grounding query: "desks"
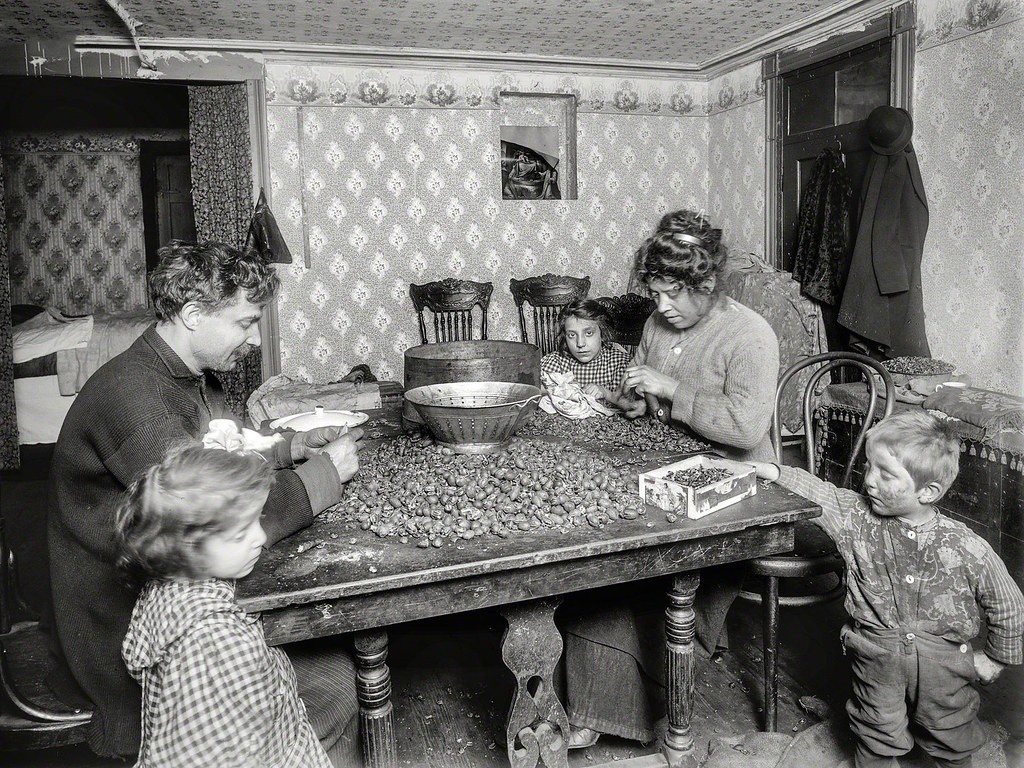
[235,402,822,768]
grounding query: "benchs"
[719,249,828,441]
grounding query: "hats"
[866,105,913,156]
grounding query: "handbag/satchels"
[242,187,292,266]
[501,140,562,200]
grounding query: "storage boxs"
[637,453,756,520]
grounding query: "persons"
[46,239,365,768]
[539,210,779,750]
[113,442,332,768]
[742,408,1024,768]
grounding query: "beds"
[10,305,160,445]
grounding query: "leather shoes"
[555,724,601,749]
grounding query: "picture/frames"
[499,91,578,200]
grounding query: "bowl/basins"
[402,381,541,455]
[881,358,956,396]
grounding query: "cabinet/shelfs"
[822,382,1024,598]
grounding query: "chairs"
[509,273,591,357]
[409,278,493,344]
[737,351,895,731]
[0,515,94,752]
[594,293,657,346]
